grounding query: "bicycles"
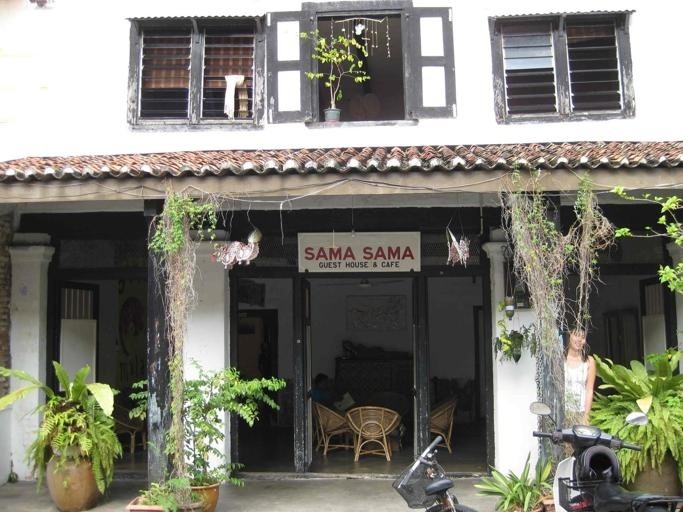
[391,434,479,512]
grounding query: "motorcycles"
[530,401,682,512]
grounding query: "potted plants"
[300,28,372,121]
[472,457,553,512]
[589,348,683,496]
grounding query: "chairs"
[312,396,458,461]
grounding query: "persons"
[308,373,353,440]
[561,322,598,459]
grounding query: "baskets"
[557,477,607,512]
[392,458,447,509]
